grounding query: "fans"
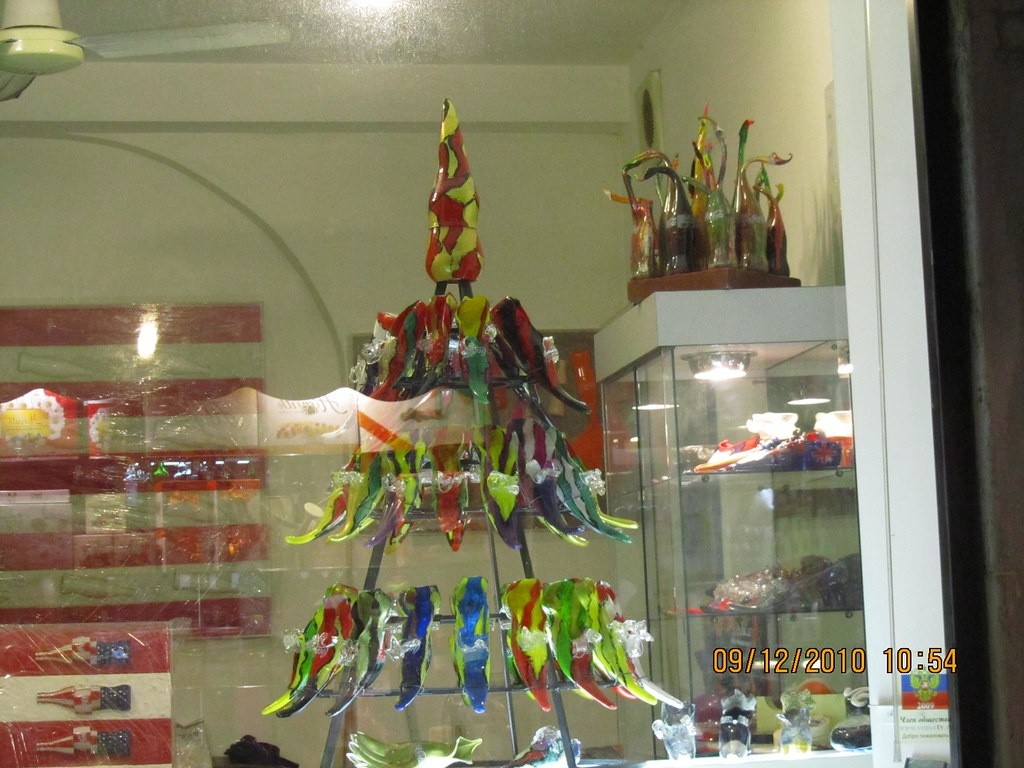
[0,0,289,105]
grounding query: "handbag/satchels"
[224,734,299,768]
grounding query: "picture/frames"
[347,328,607,532]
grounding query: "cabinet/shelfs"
[589,283,875,768]
